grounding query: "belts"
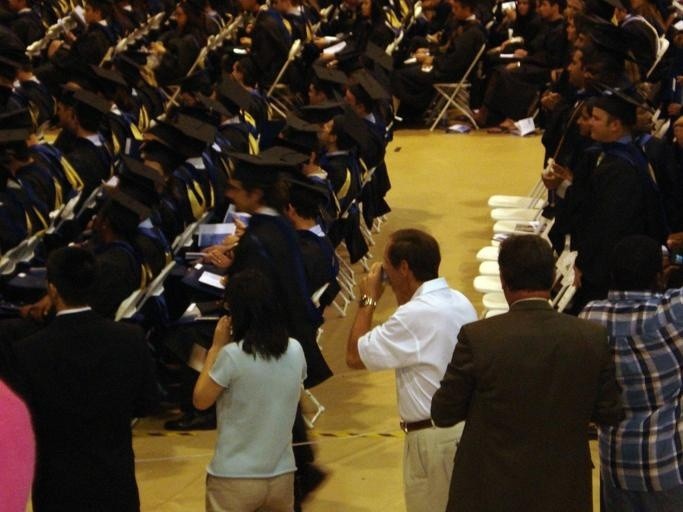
[400,414,434,433]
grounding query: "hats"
[573,0,655,126]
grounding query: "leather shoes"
[164,411,217,431]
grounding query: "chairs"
[473,176,578,320]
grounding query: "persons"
[575,236,683,511]
[390,0,528,133]
[0,2,394,512]
[530,1,682,434]
[342,228,480,512]
[429,234,624,511]
